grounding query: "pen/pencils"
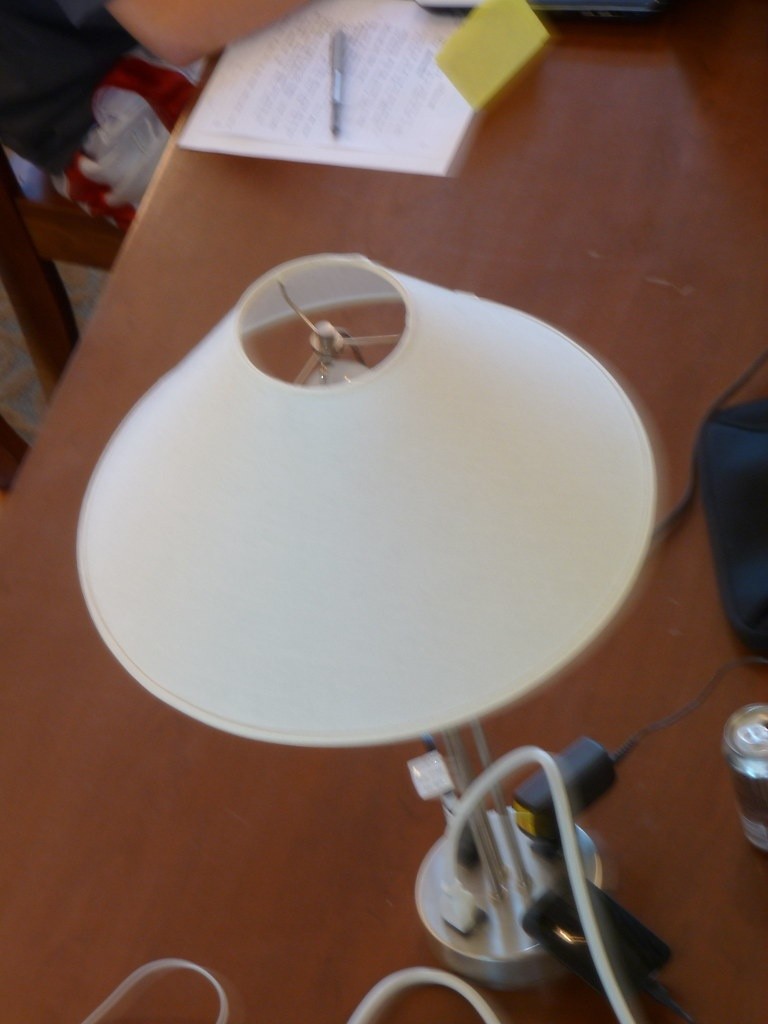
[329,30,345,139]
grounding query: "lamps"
[73,248,662,990]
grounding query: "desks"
[0,0,768,1024]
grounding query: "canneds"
[722,703,768,853]
